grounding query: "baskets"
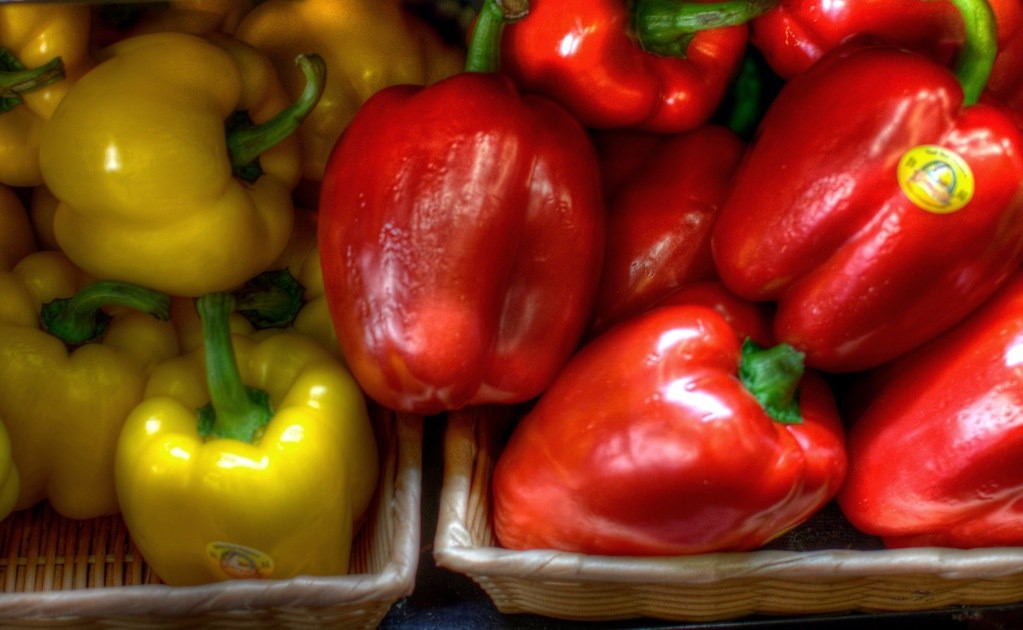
[0,401,422,630]
[432,401,1023,618]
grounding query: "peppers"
[0,0,1023,588]
